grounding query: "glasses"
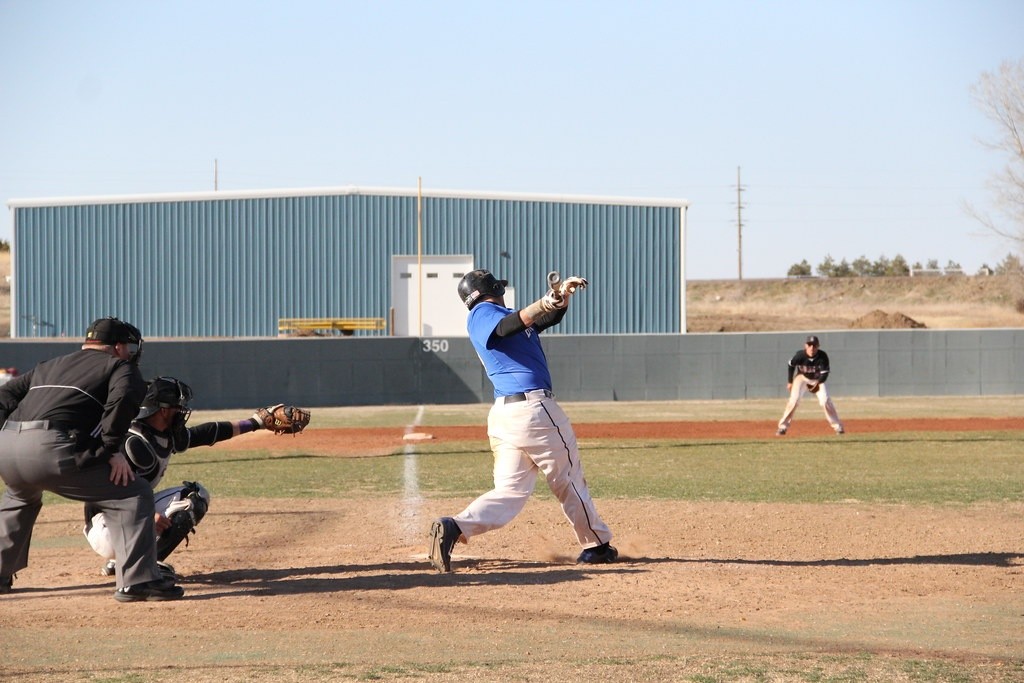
[807,342,819,348]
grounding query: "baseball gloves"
[255,402,312,435]
[806,383,819,393]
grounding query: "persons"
[1,317,183,603]
[83,376,311,577]
[776,336,844,435]
[430,270,619,571]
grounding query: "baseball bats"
[547,271,561,299]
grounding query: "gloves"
[542,289,563,316]
[557,274,588,299]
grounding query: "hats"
[86,318,143,345]
[134,375,190,419]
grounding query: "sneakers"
[0,576,14,595]
[153,561,175,578]
[103,559,116,576]
[429,517,460,573]
[114,580,186,603]
[577,541,619,567]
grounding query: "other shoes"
[835,428,845,436]
[776,428,785,436]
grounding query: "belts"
[500,390,552,404]
[3,420,70,437]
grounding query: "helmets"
[805,336,820,344]
[456,269,509,310]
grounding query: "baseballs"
[715,295,723,301]
